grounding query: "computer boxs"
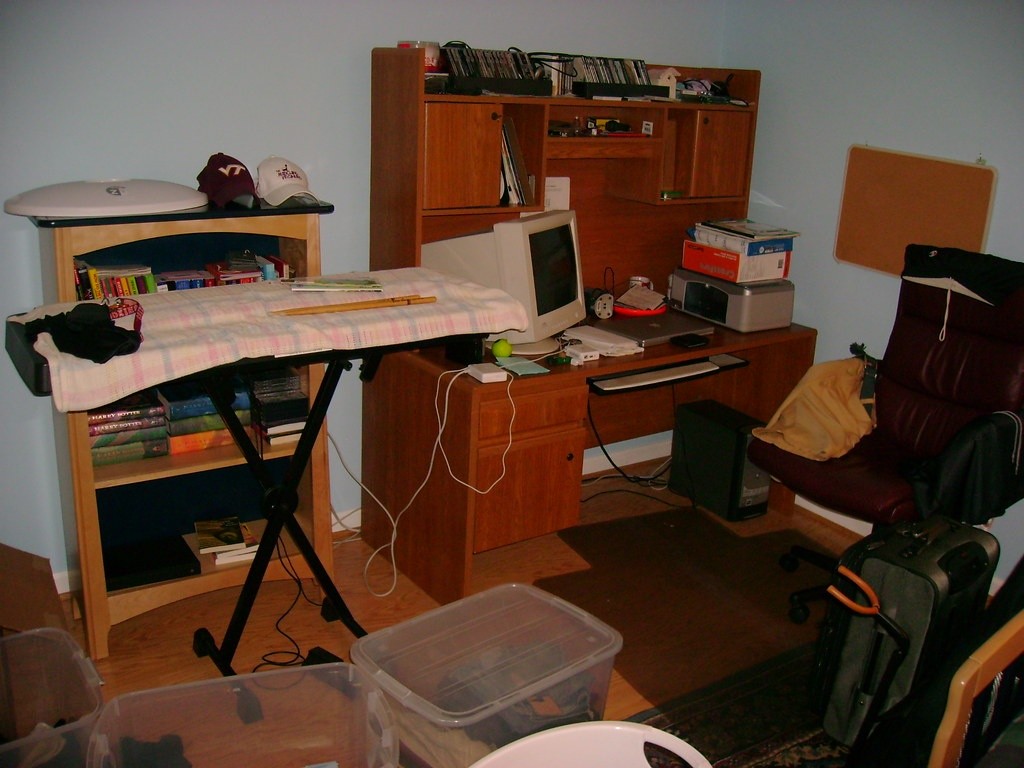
[668,398,773,523]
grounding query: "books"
[194,519,259,566]
[88,393,168,467]
[424,73,449,76]
[682,217,801,283]
[74,254,296,301]
[291,277,383,292]
[499,115,535,207]
[592,96,622,101]
[440,47,536,79]
[570,55,651,86]
[155,361,310,456]
[624,94,673,102]
[550,54,572,97]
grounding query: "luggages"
[824,516,1000,756]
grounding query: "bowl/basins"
[397,40,442,72]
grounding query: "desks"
[6,267,527,722]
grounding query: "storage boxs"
[0,626,106,768]
[84,660,400,768]
[349,584,625,767]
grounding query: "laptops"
[593,310,715,347]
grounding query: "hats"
[256,155,320,206]
[197,152,262,205]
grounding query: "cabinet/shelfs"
[22,197,334,667]
[358,44,821,604]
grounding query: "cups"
[629,276,653,291]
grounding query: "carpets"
[537,504,833,713]
[613,639,929,768]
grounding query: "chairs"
[753,243,1024,542]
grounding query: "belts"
[859,351,877,417]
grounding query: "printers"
[669,265,795,333]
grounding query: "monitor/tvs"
[420,210,586,353]
